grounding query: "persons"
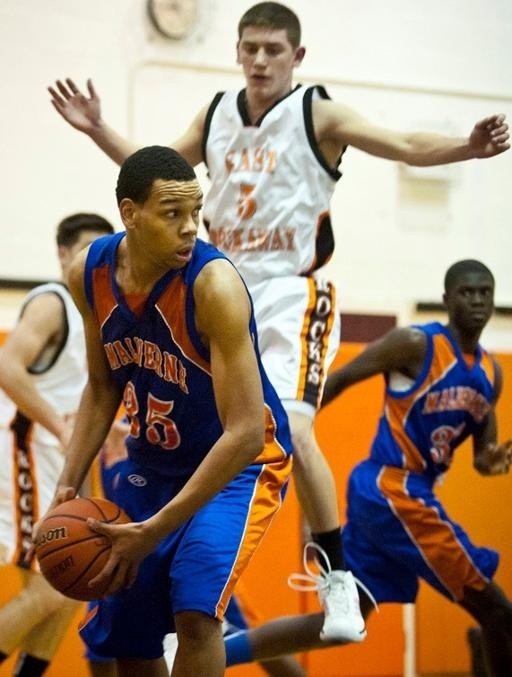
[47,2,510,647]
[221,259,510,677]
[27,143,294,676]
[0,214,117,676]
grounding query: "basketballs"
[36,499,133,599]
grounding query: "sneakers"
[319,569,367,643]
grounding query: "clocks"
[150,1,200,38]
